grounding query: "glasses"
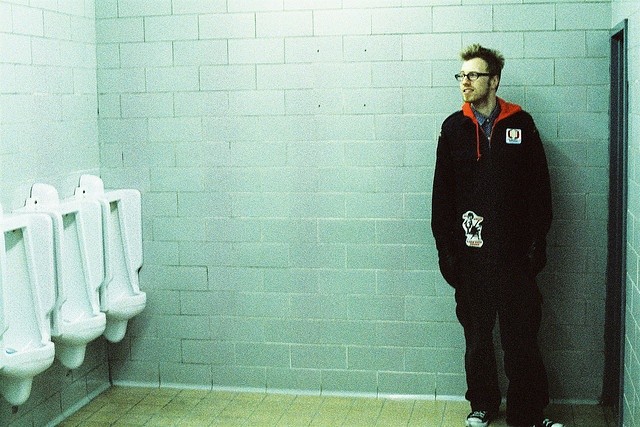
[455,71,491,81]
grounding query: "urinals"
[1,213,55,406]
[89,188,147,344]
[38,201,106,369]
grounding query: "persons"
[431,44,565,427]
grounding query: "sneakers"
[465,410,493,427]
[532,417,564,427]
